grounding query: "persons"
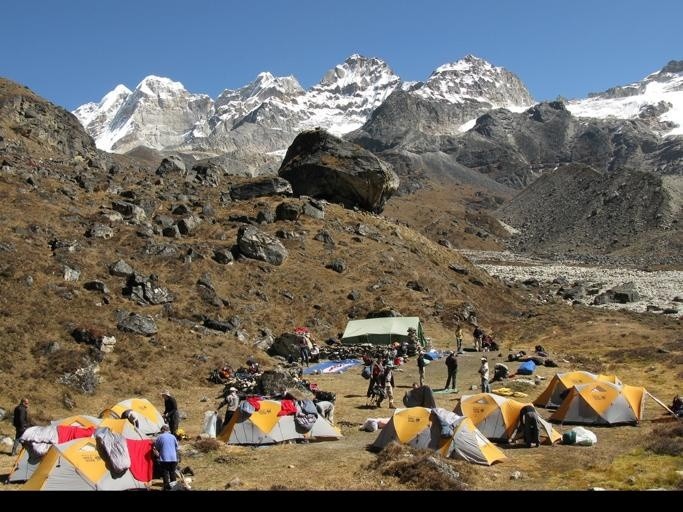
[473,325,483,351]
[11,397,31,457]
[365,355,397,409]
[153,425,177,491]
[444,351,458,389]
[416,349,427,387]
[520,406,539,448]
[478,355,490,392]
[161,388,180,438]
[222,386,237,424]
[454,324,463,350]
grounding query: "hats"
[161,390,171,397]
[230,387,238,391]
[479,358,486,361]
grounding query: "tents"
[218,399,345,442]
[101,397,168,437]
[453,390,562,447]
[532,370,622,408]
[21,417,153,490]
[546,380,648,424]
[5,413,102,483]
[370,406,506,466]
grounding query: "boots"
[388,399,397,409]
[376,398,383,407]
